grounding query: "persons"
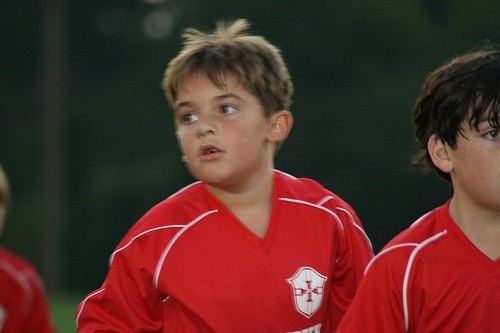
[0,167,56,333]
[75,20,374,333]
[335,50,500,333]
